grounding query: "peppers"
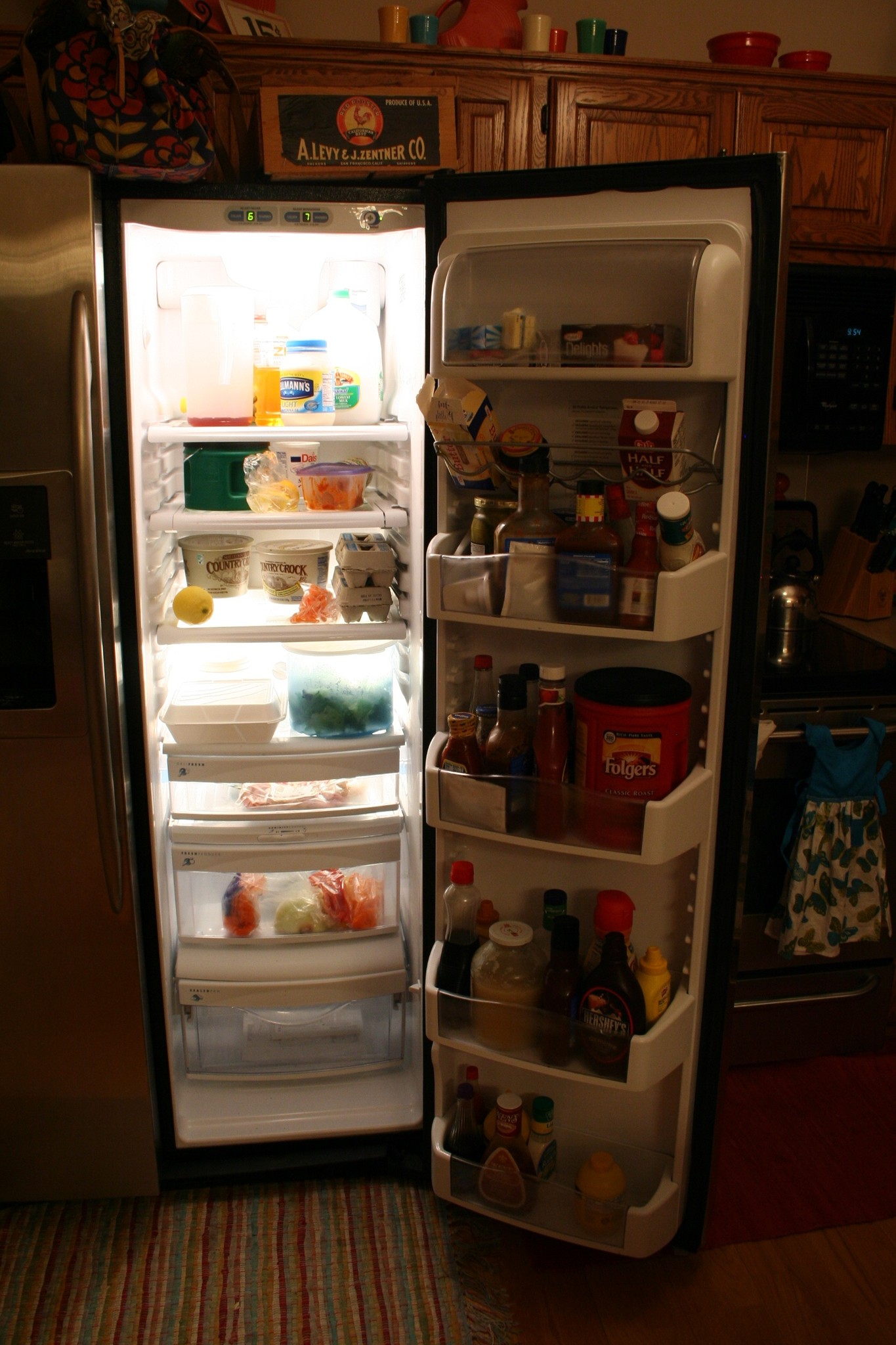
[222,870,265,935]
[307,867,378,930]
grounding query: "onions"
[276,898,326,935]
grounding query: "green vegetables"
[289,679,393,737]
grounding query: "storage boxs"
[157,682,291,746]
[559,324,682,368]
[457,325,500,350]
[419,373,502,491]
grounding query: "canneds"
[499,424,555,494]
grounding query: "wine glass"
[616,500,662,630]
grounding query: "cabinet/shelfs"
[201,32,896,258]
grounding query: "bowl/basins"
[778,50,832,72]
[705,31,782,67]
[178,533,255,598]
[296,463,375,510]
[252,539,333,605]
[157,678,290,744]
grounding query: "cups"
[521,14,553,52]
[549,27,568,52]
[603,28,628,56]
[377,5,409,43]
[409,14,438,46]
[576,18,607,55]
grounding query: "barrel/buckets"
[180,442,270,511]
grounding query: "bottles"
[531,662,575,842]
[0,149,794,1283]
[442,1065,629,1238]
[553,479,624,626]
[657,491,705,573]
[252,312,285,427]
[303,288,383,425]
[572,665,696,851]
[492,455,565,617]
[470,493,519,557]
[439,654,540,831]
[272,441,320,509]
[432,857,671,1084]
[279,339,336,426]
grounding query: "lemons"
[172,586,214,625]
